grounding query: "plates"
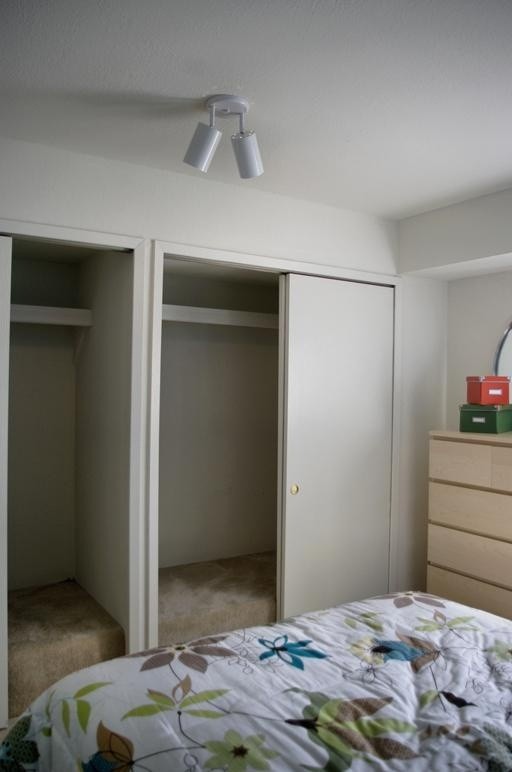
[0,217,401,728]
[424,431,511,621]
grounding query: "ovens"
[24,591,512,771]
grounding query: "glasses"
[181,92,265,181]
